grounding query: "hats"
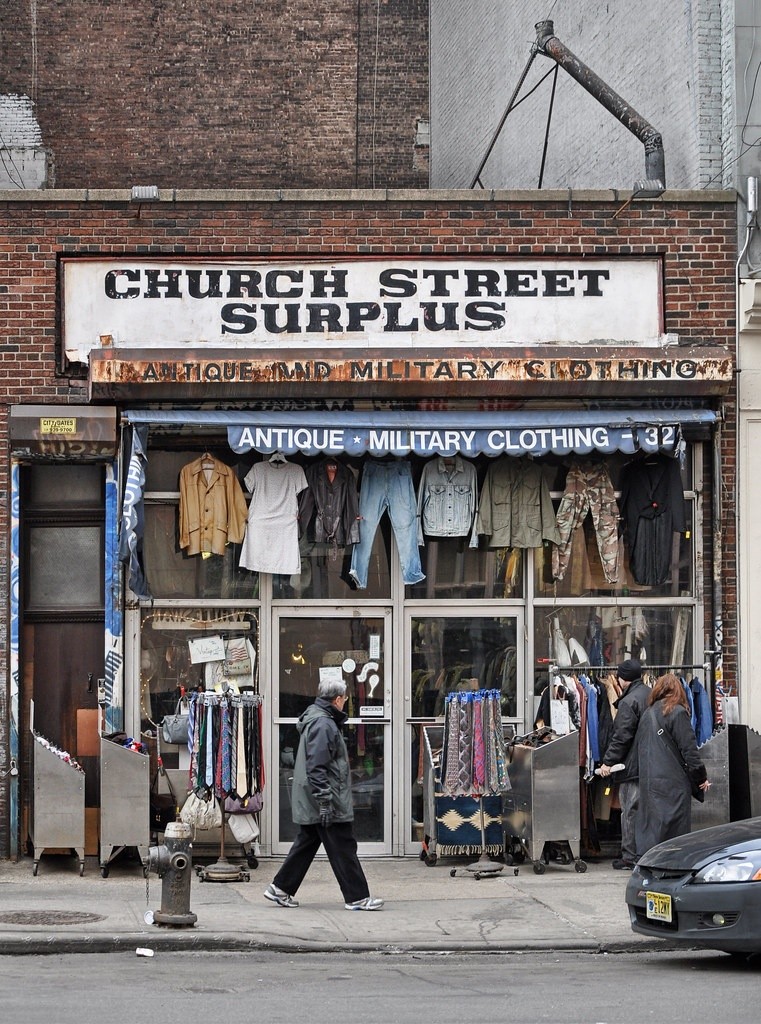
[618,659,641,682]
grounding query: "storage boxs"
[176,813,251,856]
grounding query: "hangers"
[201,444,214,461]
[558,666,693,684]
[269,451,287,463]
[645,452,659,465]
[323,456,338,462]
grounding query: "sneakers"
[344,897,385,911]
[263,883,299,908]
[612,859,636,870]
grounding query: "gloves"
[319,803,337,828]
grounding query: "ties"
[440,691,513,803]
[365,758,373,777]
[189,697,266,809]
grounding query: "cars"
[625,815,761,973]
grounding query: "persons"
[601,659,710,871]
[264,677,384,911]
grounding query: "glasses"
[344,695,348,701]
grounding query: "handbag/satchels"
[223,637,252,675]
[225,792,264,812]
[553,630,571,667]
[228,814,260,844]
[688,770,705,803]
[150,768,177,833]
[162,696,191,744]
[568,637,590,667]
[179,789,222,829]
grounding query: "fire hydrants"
[141,818,198,929]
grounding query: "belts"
[343,674,366,751]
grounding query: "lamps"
[130,185,160,220]
[612,179,666,218]
